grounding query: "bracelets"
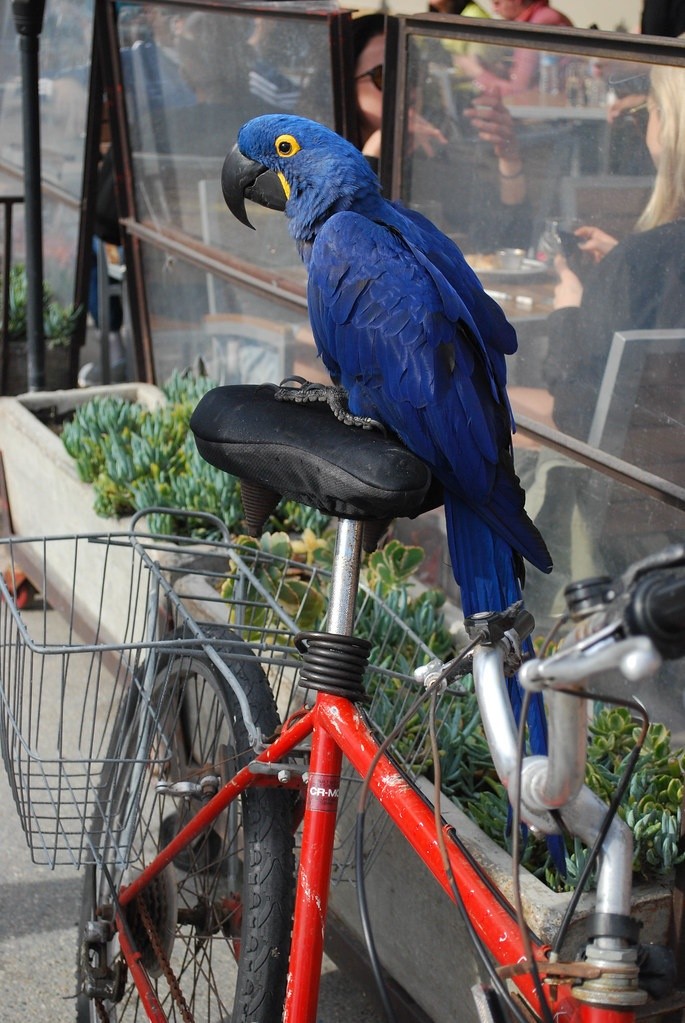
[498,159,525,179]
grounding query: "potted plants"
[0,369,685,1023]
[0,262,85,395]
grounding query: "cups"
[496,248,526,270]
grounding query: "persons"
[409,0,500,94]
[37,1,345,326]
[290,9,533,252]
[505,65,685,454]
[454,0,587,93]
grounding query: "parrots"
[220,113,574,884]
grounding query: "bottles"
[536,215,586,263]
[539,50,561,96]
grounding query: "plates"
[463,255,546,276]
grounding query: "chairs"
[198,177,296,382]
[560,174,657,232]
[577,327,685,543]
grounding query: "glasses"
[357,58,430,92]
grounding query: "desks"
[274,255,560,382]
[500,88,633,175]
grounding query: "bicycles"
[74,382,685,1023]
